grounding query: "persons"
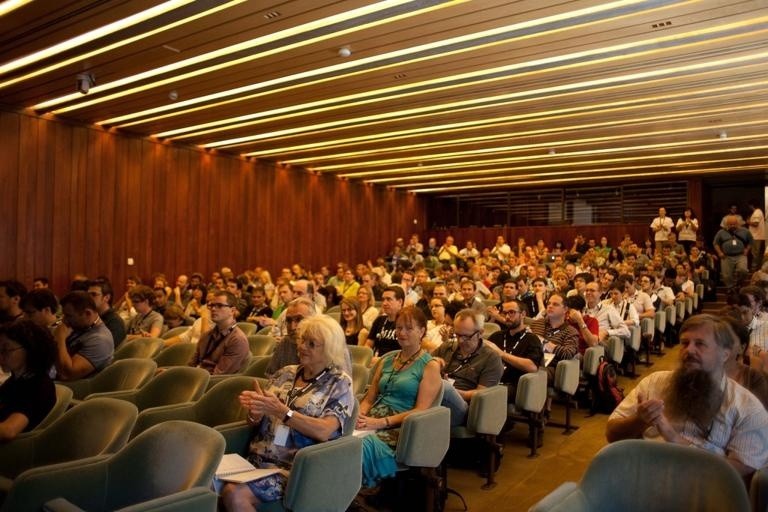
[0,202,768,512]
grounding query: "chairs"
[0,252,768,512]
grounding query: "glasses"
[207,302,231,308]
[454,330,477,339]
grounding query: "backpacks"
[593,355,624,415]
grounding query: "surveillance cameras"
[76,75,90,95]
